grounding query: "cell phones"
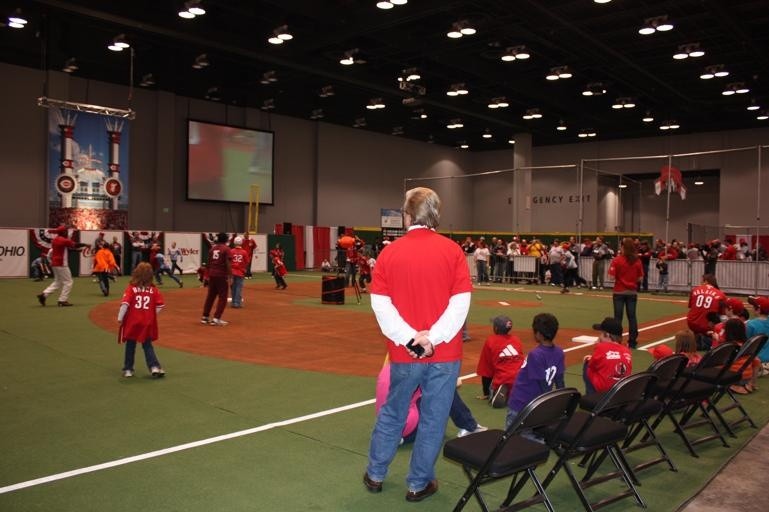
[406,338,425,358]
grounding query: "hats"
[748,295,769,314]
[592,317,623,335]
[720,298,744,312]
[647,345,673,361]
[489,315,513,330]
[234,236,242,245]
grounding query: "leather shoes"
[406,479,438,502]
[364,472,382,493]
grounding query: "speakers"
[284,222,291,235]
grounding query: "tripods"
[345,265,361,304]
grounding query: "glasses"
[400,207,407,214]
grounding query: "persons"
[449,235,768,294]
[30,226,287,326]
[364,186,475,501]
[374,349,490,445]
[582,317,633,394]
[606,237,645,349]
[646,295,769,413]
[116,262,167,378]
[504,313,567,446]
[476,315,527,410]
[335,235,402,295]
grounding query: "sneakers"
[729,384,748,394]
[200,316,208,324]
[125,370,132,377]
[457,424,488,438]
[58,301,73,306]
[210,318,228,326]
[745,383,752,392]
[491,384,508,408]
[37,293,46,306]
[179,281,183,288]
[151,366,165,376]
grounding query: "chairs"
[643,340,741,457]
[531,371,659,511]
[582,353,689,485]
[690,332,767,439]
[443,386,580,511]
